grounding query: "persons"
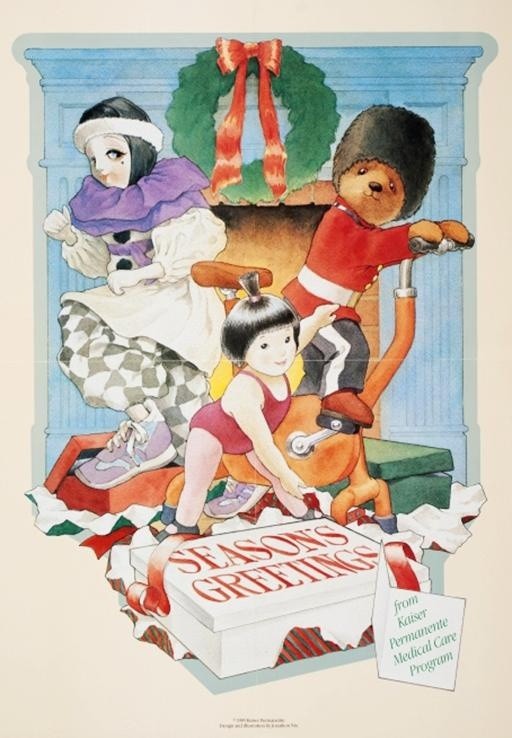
[43,96,271,518]
[153,271,338,542]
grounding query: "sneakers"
[75,408,178,489]
[203,477,272,519]
[320,390,374,429]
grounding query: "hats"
[72,98,164,153]
